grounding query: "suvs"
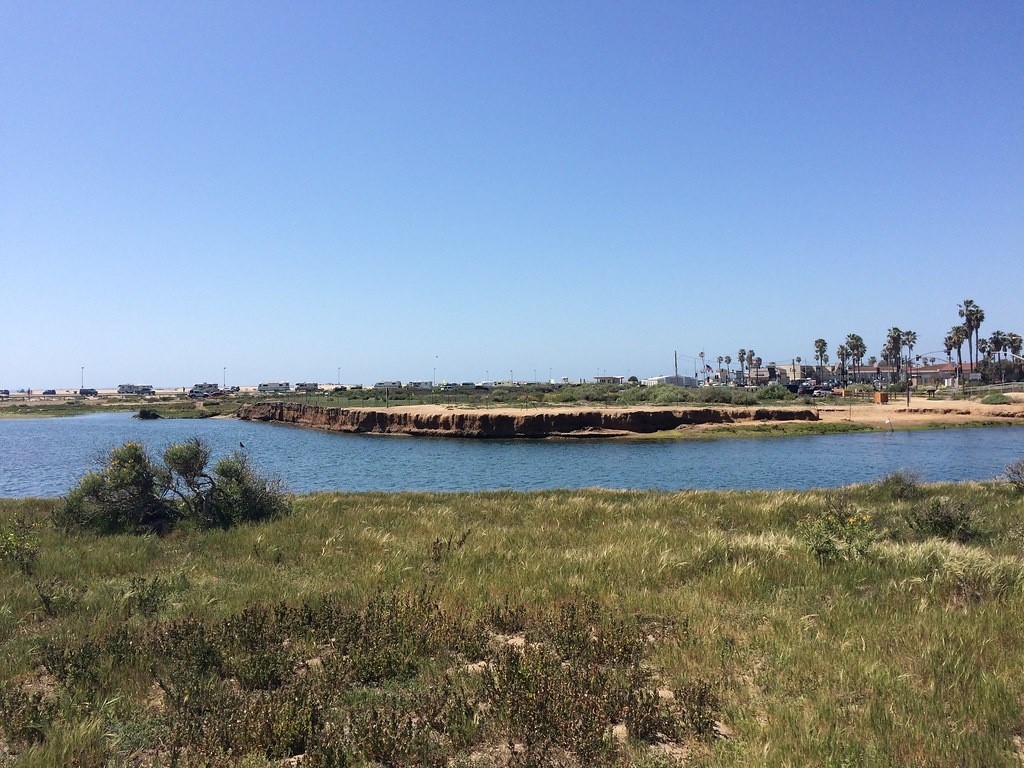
[189,390,210,399]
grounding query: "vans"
[440,385,456,392]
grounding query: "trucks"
[259,381,319,393]
[80,389,98,397]
[117,384,156,397]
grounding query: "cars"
[786,376,890,398]
[212,385,240,396]
[43,389,57,395]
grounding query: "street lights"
[338,367,341,385]
[81,366,85,389]
[224,366,226,387]
[486,370,489,382]
[550,367,552,381]
[510,369,513,384]
[433,367,436,386]
[534,370,537,385]
